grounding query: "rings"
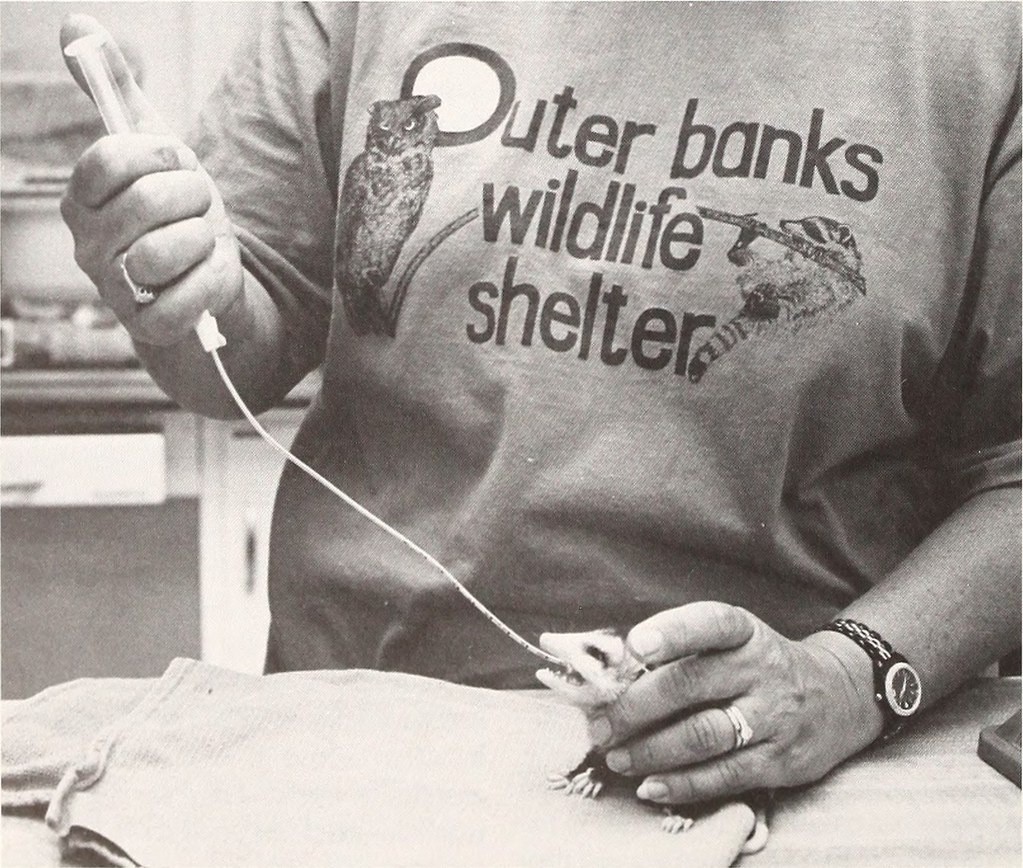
[121,254,155,304]
[724,706,752,749]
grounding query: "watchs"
[818,619,922,746]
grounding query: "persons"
[59,0,1023,807]
